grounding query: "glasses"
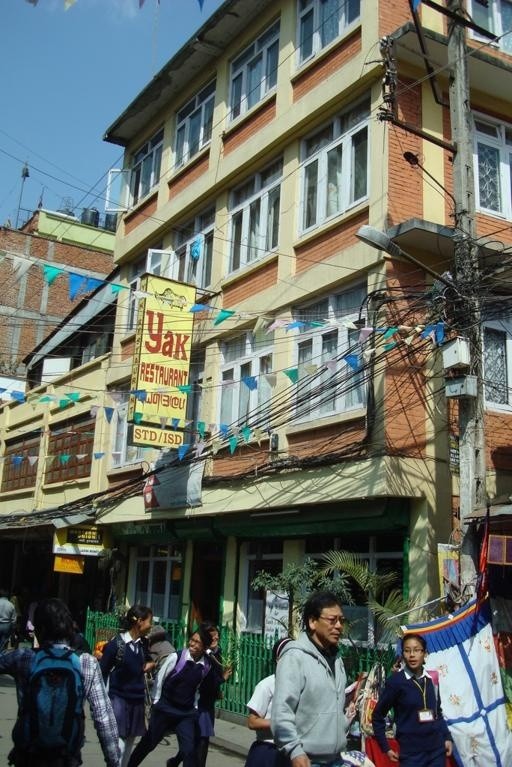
[402,649,426,654]
[318,614,345,626]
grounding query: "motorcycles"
[97,616,178,679]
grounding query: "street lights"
[356,218,479,614]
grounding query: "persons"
[372,634,454,767]
[270,591,347,767]
[243,638,295,767]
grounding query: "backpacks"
[16,646,85,766]
